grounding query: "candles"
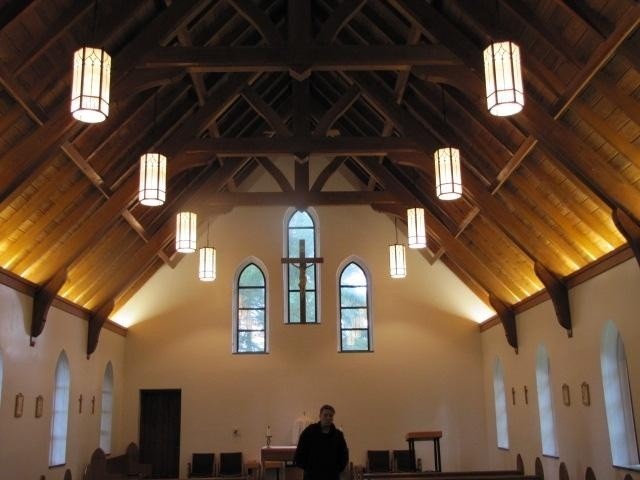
[267,424,271,436]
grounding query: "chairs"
[187,452,215,480]
[392,450,422,473]
[216,451,244,478]
[365,449,392,473]
[90,448,126,479]
[126,443,154,477]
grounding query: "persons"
[290,262,314,295]
[292,404,349,479]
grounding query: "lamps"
[198,218,217,284]
[175,167,198,255]
[388,215,407,280]
[138,87,166,207]
[407,162,427,248]
[69,1,113,125]
[431,85,463,201]
[479,1,525,118]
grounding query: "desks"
[260,445,298,479]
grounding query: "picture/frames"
[13,392,25,418]
[581,382,590,407]
[35,393,43,418]
[562,384,570,407]
